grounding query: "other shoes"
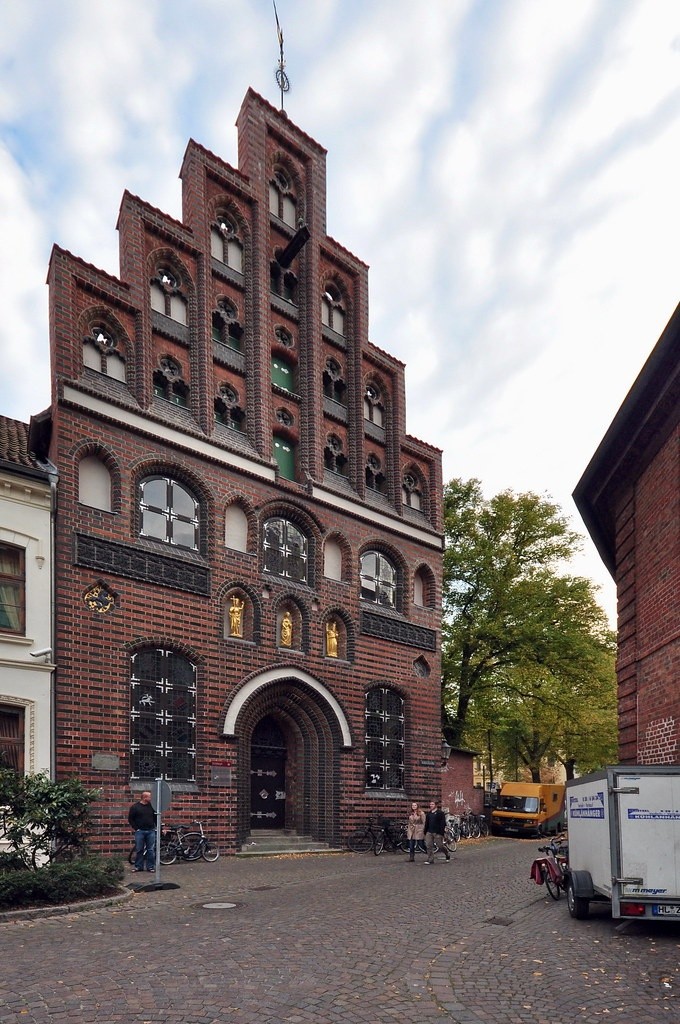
[424,861,433,865]
[405,859,415,862]
[445,855,451,862]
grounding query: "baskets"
[377,818,390,826]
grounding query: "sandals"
[147,867,155,873]
[131,867,143,872]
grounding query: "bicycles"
[536,832,568,902]
[127,818,220,866]
[347,810,489,856]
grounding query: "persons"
[406,802,427,861]
[128,792,157,872]
[424,800,450,864]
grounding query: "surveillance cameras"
[29,648,52,657]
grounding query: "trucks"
[490,782,567,839]
[561,763,680,927]
